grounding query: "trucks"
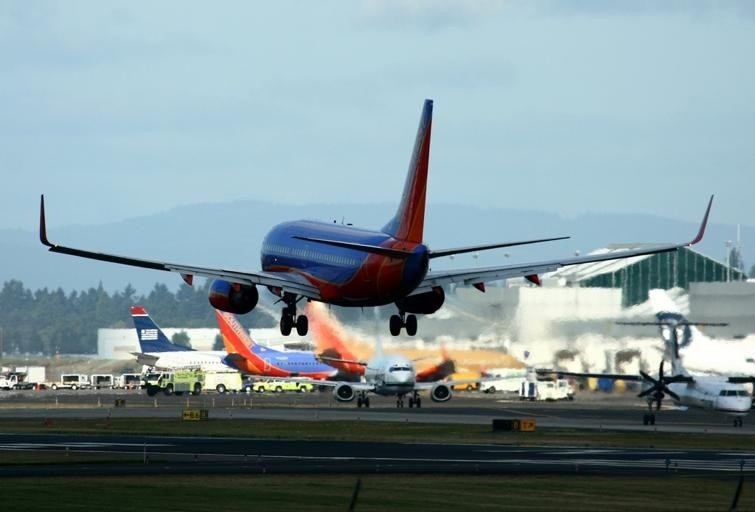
[0,369,206,396]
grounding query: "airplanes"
[532,311,753,428]
[39,99,715,337]
